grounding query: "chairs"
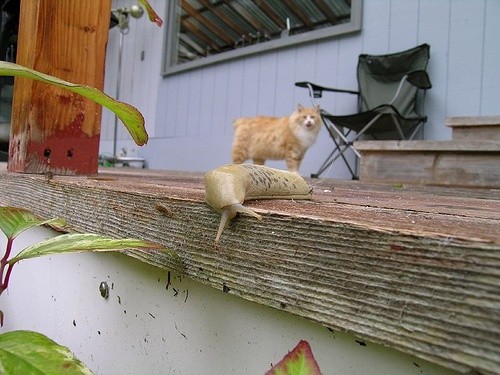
[294,43,430,180]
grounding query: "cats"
[232,103,322,175]
